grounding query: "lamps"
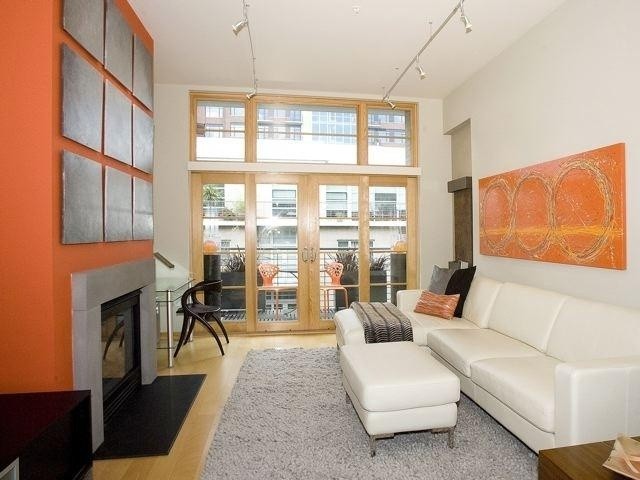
[380,0,472,110]
[153,251,174,269]
[233,1,258,100]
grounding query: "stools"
[340,341,460,455]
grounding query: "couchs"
[333,255,637,456]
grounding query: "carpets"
[92,373,208,460]
[195,346,517,480]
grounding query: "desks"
[155,275,196,368]
[531,437,639,480]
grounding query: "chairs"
[174,279,229,356]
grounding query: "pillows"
[415,264,478,321]
